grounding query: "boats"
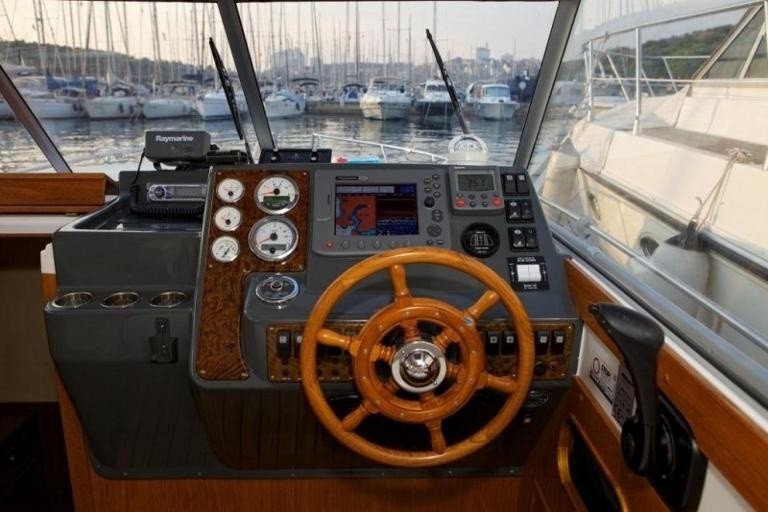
[534,0,768,390]
